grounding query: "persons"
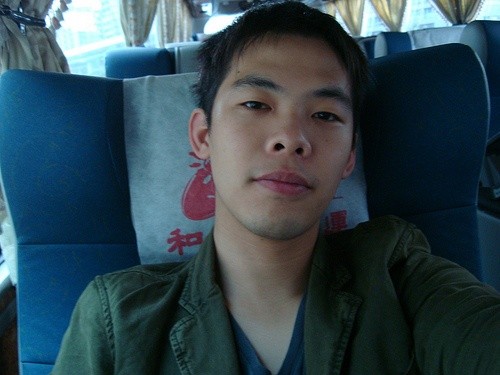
[50,0,500,375]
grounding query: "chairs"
[0,22,500,375]
